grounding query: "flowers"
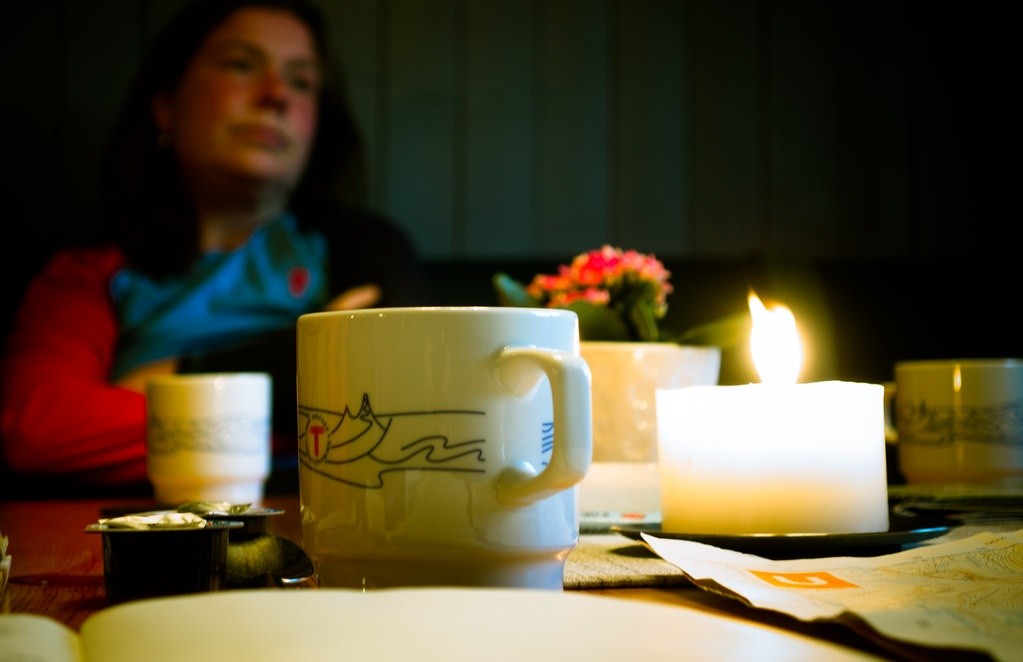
[528,243,674,340]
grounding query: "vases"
[575,342,722,522]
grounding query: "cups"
[144,371,274,509]
[295,304,593,591]
[884,358,1023,496]
[83,519,243,600]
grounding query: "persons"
[0,2,479,501]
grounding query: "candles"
[656,288,890,533]
